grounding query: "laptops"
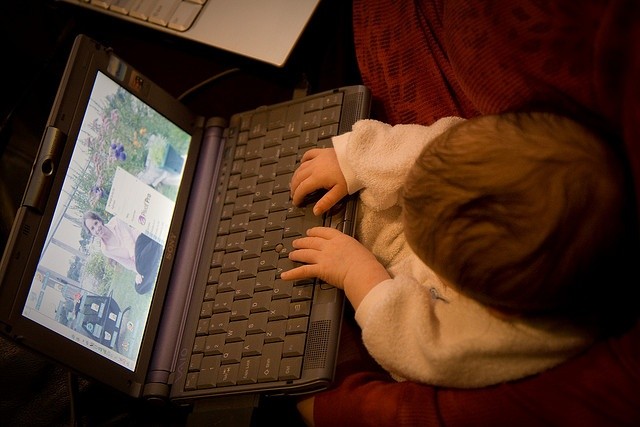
[0,33,371,408]
[67,0,322,67]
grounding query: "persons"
[83,211,144,286]
[282,110,639,389]
